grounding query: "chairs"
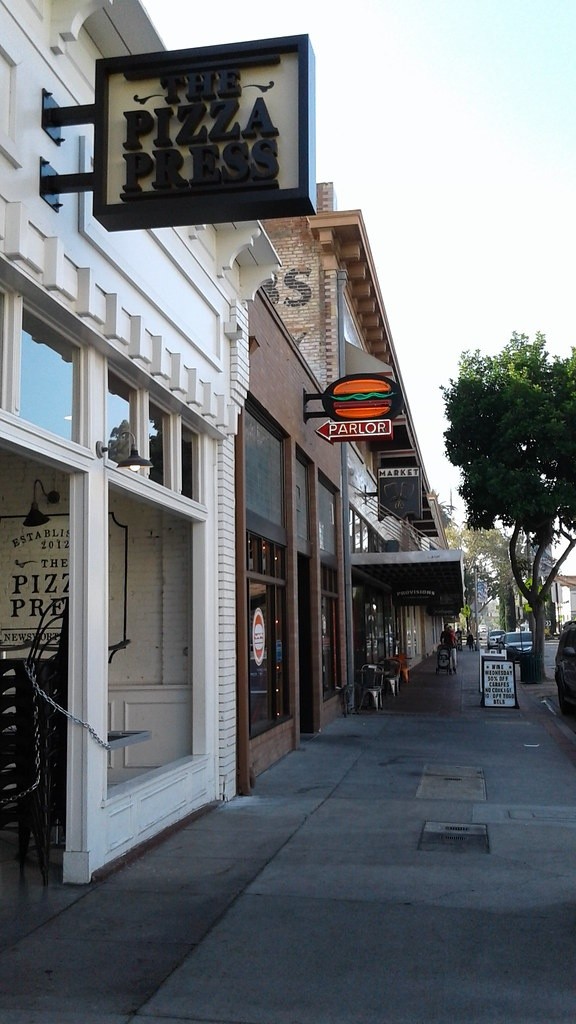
[354,658,400,713]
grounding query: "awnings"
[347,343,439,538]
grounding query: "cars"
[481,627,486,633]
[488,630,506,650]
[497,631,533,661]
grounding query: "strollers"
[436,645,452,676]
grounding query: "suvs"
[555,620,576,714]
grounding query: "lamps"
[95,430,154,471]
[22,479,60,527]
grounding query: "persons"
[440,624,475,674]
